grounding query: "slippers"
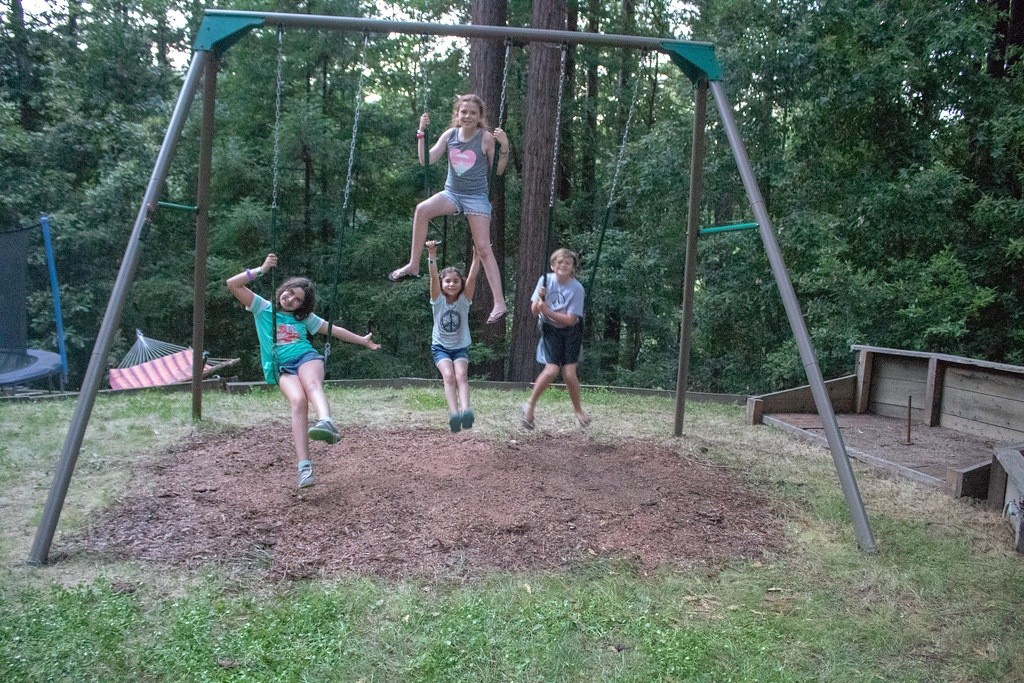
[486,310,509,326]
[388,268,425,282]
[578,415,591,428]
[523,411,535,431]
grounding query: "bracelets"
[499,146,509,155]
[417,129,425,138]
[245,268,255,280]
[255,266,263,277]
[427,256,438,262]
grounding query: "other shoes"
[462,410,474,429]
[449,414,461,433]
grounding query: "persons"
[520,247,593,429]
[424,235,480,433]
[227,251,381,487]
[389,93,510,326]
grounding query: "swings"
[531,39,650,365]
[269,21,371,389]
[416,29,512,207]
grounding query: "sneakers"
[307,419,342,444]
[297,462,316,489]
